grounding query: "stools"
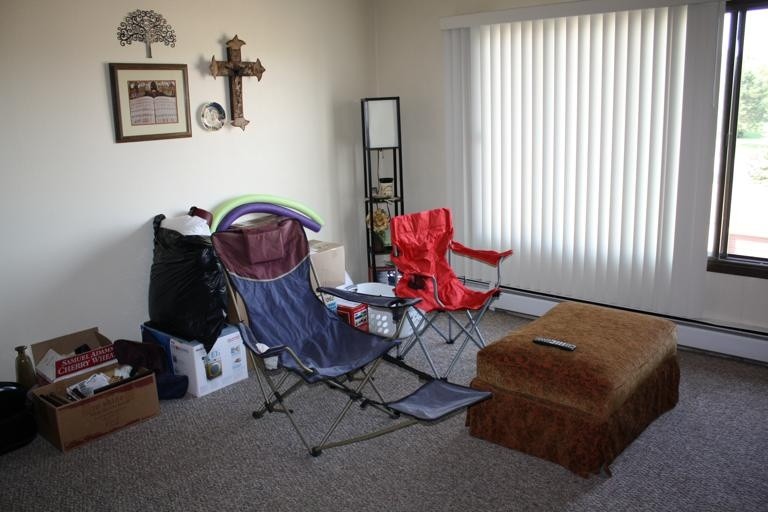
[465,300,680,480]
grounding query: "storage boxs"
[22,321,251,449]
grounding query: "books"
[22,321,251,449]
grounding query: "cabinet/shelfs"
[358,93,405,285]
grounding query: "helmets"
[200,101,226,131]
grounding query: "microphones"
[465,300,680,480]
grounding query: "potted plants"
[107,61,194,144]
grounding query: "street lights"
[0,381,37,456]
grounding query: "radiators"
[532,337,576,352]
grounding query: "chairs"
[391,209,513,384]
[211,218,493,457]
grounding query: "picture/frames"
[107,61,194,144]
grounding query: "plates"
[358,93,405,285]
